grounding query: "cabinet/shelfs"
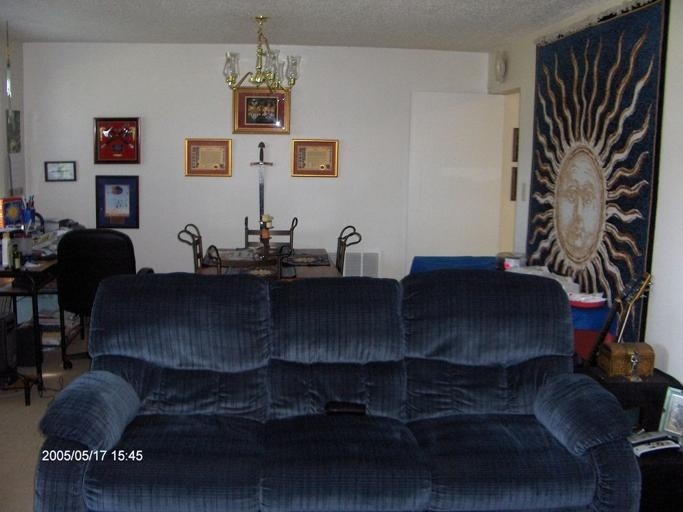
[0,255,72,407]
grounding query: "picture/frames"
[185,88,337,177]
[45,117,140,229]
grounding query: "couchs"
[34,268,643,511]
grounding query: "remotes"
[626,431,668,443]
[633,440,680,458]
[325,401,365,416]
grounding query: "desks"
[203,246,331,268]
[585,364,683,511]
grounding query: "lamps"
[223,14,297,90]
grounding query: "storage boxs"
[598,342,655,377]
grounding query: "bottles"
[0,232,21,272]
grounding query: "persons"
[106,186,127,214]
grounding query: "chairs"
[179,223,203,273]
[257,245,296,279]
[241,217,298,248]
[335,226,361,277]
[56,230,136,341]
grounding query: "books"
[38,309,80,346]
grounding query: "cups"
[23,207,36,224]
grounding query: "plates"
[569,298,607,309]
